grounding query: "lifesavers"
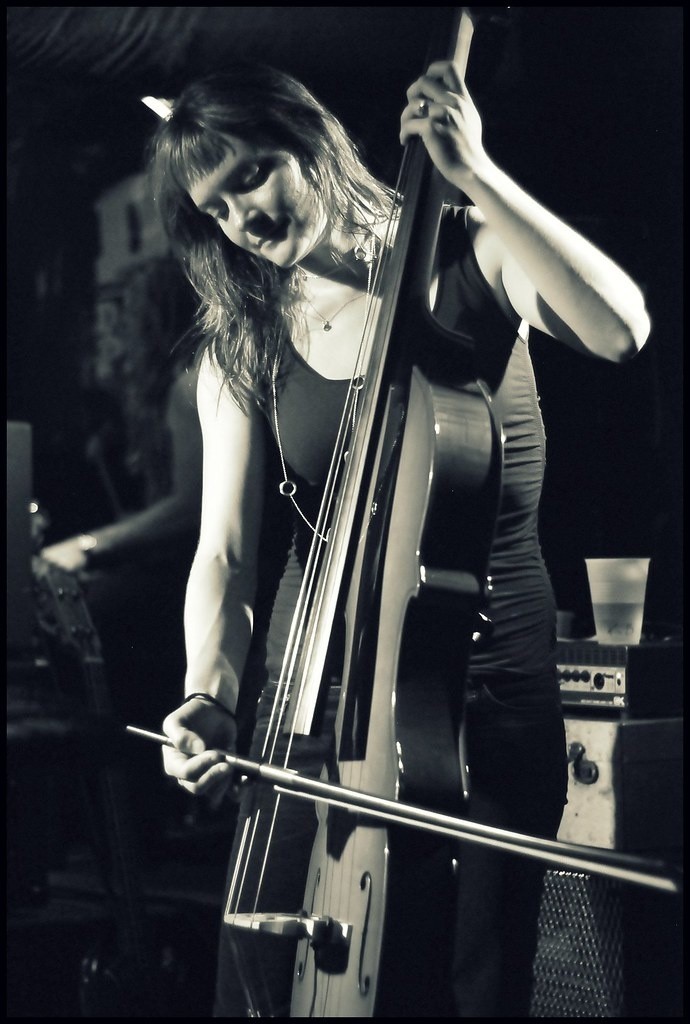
[207,72,472,1022]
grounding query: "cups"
[584,559,651,644]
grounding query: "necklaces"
[295,216,383,331]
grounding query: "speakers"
[530,867,684,1017]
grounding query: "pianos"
[1,412,126,755]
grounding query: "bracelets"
[184,692,240,727]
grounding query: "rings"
[418,99,428,117]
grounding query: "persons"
[152,61,650,1016]
